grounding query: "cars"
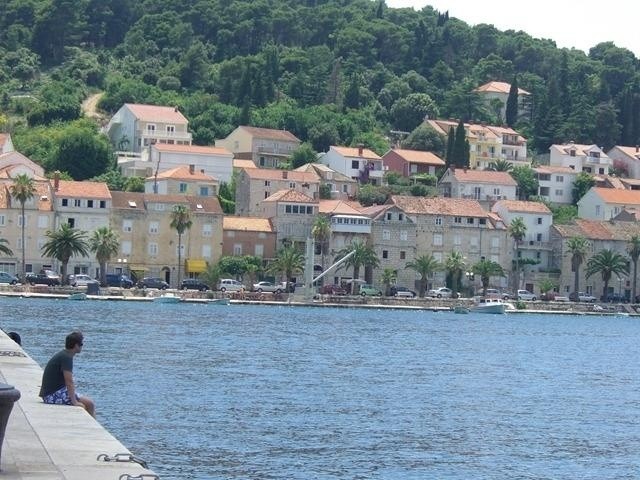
[36,270,62,286]
[17,272,37,282]
[181,279,210,292]
[0,272,18,285]
[319,284,348,295]
[393,287,416,298]
[502,290,640,303]
[252,281,305,293]
[478,289,509,300]
[137,277,170,290]
[428,288,461,298]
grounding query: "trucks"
[105,273,133,289]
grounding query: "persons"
[39,331,95,419]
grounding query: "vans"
[217,279,246,292]
[69,275,101,287]
[359,284,382,296]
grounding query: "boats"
[469,297,505,314]
